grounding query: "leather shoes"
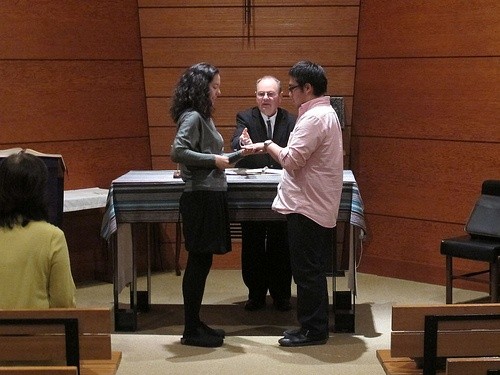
[283,326,302,336]
[279,329,327,347]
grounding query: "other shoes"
[180,323,225,347]
[275,298,293,311]
[200,321,225,338]
[244,299,266,311]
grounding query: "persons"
[0,153,77,308]
[243,61,342,346]
[169,63,259,347]
[231,76,292,311]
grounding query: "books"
[0,148,66,170]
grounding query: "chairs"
[440,180,500,304]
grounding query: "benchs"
[0,307,123,375]
[376,304,500,375]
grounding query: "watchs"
[264,140,272,151]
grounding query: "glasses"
[258,91,278,98]
[289,85,300,93]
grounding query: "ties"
[267,119,272,140]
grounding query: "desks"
[62,187,108,213]
[99,170,366,332]
